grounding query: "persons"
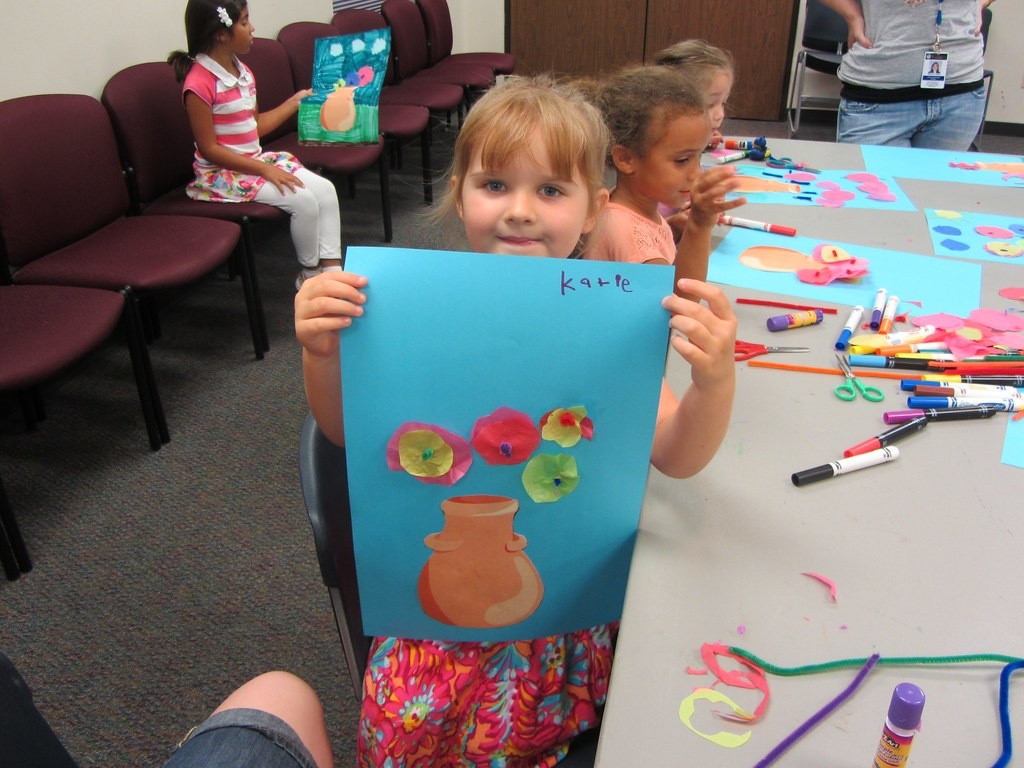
[814,0,998,152]
[562,62,747,317]
[163,670,336,768]
[928,62,940,73]
[295,53,737,768]
[168,0,343,291]
[657,40,733,138]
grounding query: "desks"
[593,134,1024,768]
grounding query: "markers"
[717,149,753,165]
[844,416,928,458]
[835,305,864,350]
[848,341,1024,376]
[908,395,1016,412]
[883,407,998,425]
[719,215,797,235]
[901,379,1017,396]
[869,288,887,331]
[878,296,898,334]
[791,445,899,483]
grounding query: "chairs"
[787,0,865,135]
[295,409,372,705]
[0,0,517,598]
[971,8,994,152]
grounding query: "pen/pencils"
[734,297,837,314]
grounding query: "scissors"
[834,353,884,401]
[734,339,811,361]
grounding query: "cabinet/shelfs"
[504,0,801,122]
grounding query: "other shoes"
[295,270,308,291]
